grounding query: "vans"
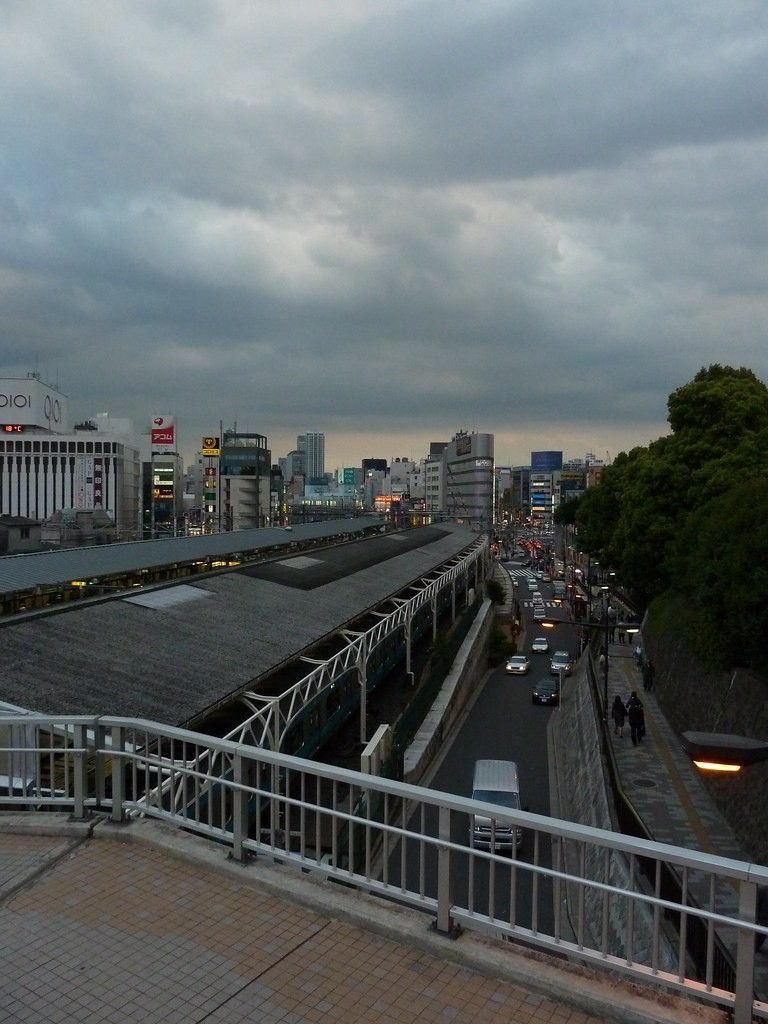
[549,650,574,677]
[532,592,542,605]
[528,579,539,591]
[465,758,532,853]
[534,606,546,624]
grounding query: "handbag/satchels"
[640,726,645,737]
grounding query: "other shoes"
[614,730,617,734]
[620,736,623,738]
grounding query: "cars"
[532,676,561,706]
[532,637,549,654]
[513,533,546,567]
[536,571,545,579]
[512,578,518,587]
[505,655,532,676]
[541,573,550,582]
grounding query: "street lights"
[553,598,592,638]
[536,596,640,719]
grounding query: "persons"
[612,696,628,737]
[628,698,644,745]
[597,589,636,644]
[642,658,654,691]
[626,691,643,735]
[634,642,644,671]
